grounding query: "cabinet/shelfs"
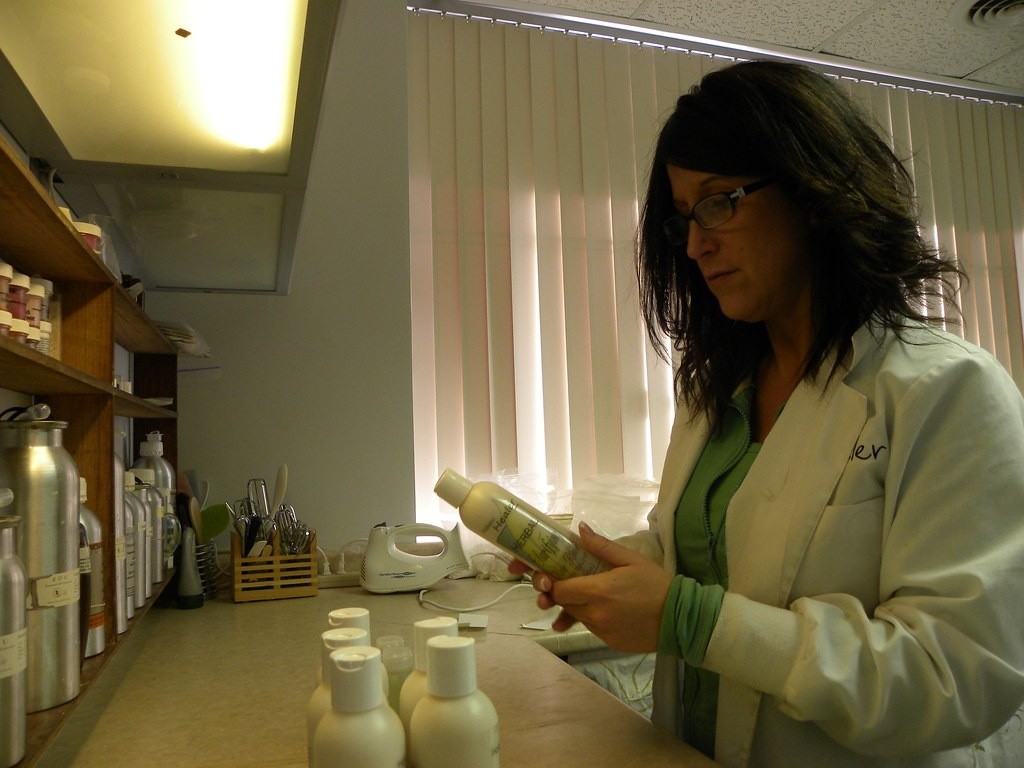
[0,139,179,768]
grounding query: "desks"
[72,549,725,768]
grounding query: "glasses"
[662,179,770,248]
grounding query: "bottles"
[0,403,105,768]
[114,428,178,634]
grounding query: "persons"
[506,55,1024,768]
